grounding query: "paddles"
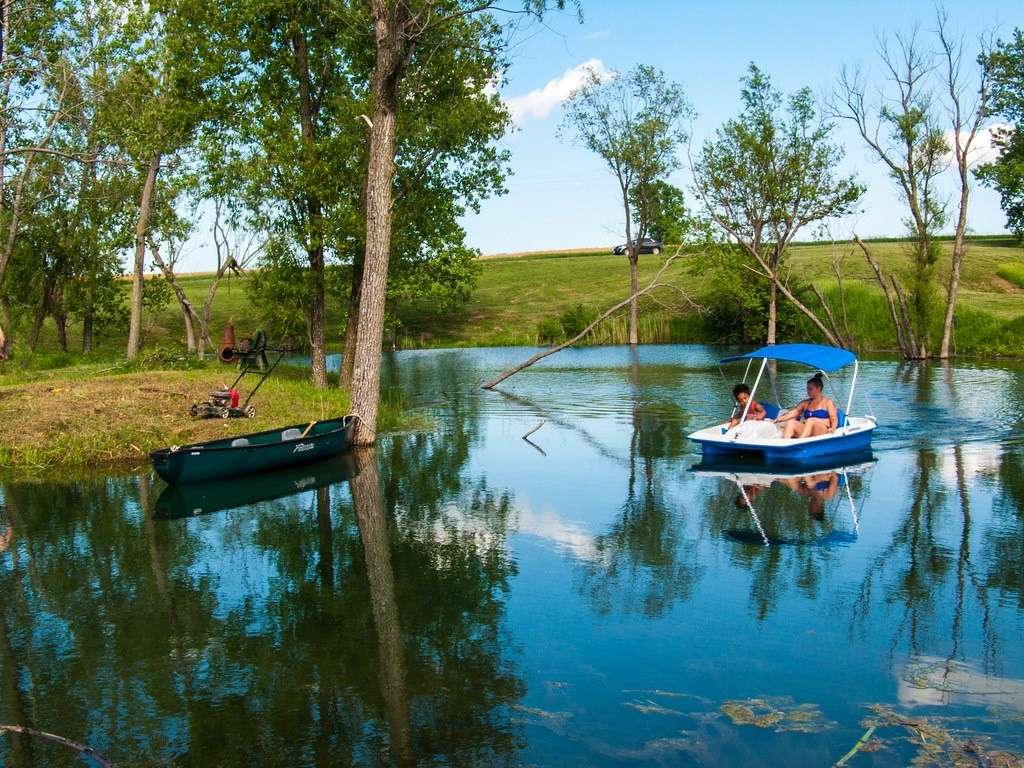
[297,420,317,439]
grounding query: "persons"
[734,470,838,521]
[721,385,767,432]
[769,373,838,439]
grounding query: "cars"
[613,238,663,256]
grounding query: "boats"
[683,449,881,547]
[150,412,362,483]
[150,451,357,521]
[681,341,878,460]
